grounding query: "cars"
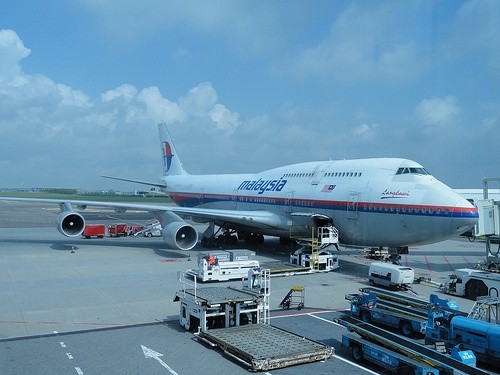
[145,229,161,238]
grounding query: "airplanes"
[0,121,480,252]
